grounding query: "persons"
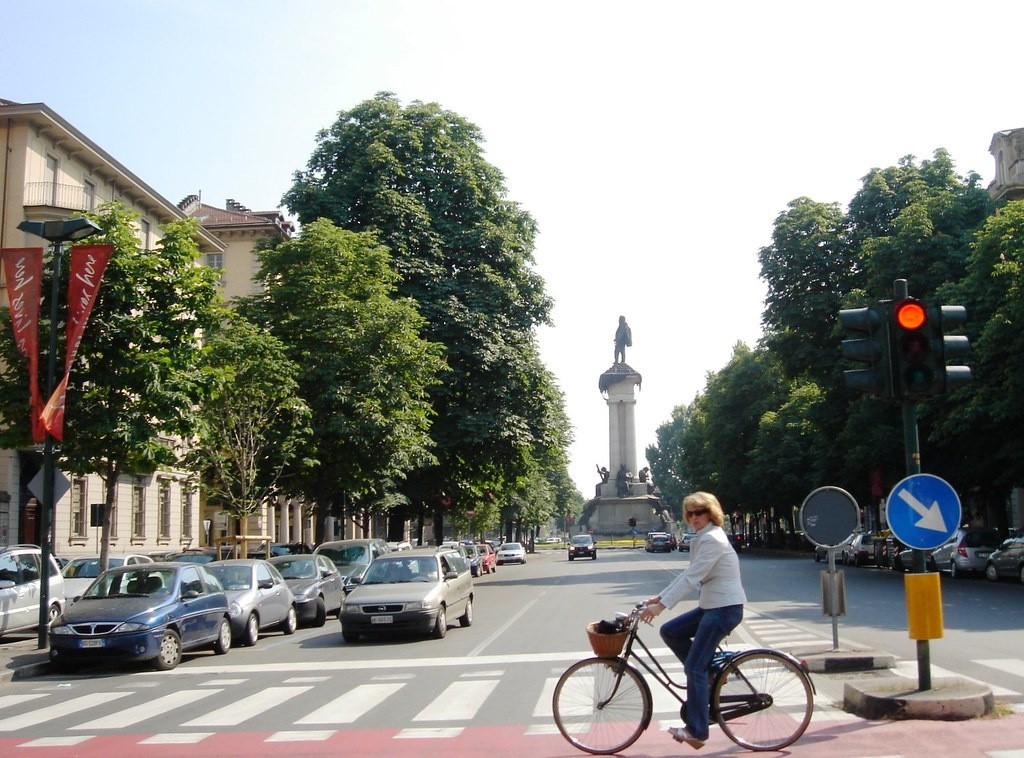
[594,467,609,499]
[633,491,747,749]
[615,464,632,499]
[639,467,654,493]
[612,316,632,364]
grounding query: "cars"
[265,554,346,627]
[813,526,1024,583]
[147,541,312,564]
[496,543,526,566]
[204,559,297,646]
[439,541,497,577]
[645,532,697,553]
[60,554,165,610]
[340,548,475,642]
[49,561,232,671]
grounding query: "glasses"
[687,509,708,516]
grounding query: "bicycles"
[552,600,816,755]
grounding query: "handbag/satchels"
[596,620,625,634]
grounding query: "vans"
[301,538,396,592]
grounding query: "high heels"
[708,669,728,688]
[668,727,706,750]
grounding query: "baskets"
[586,622,629,658]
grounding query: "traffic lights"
[888,298,936,400]
[930,300,971,394]
[839,301,891,403]
[628,518,636,526]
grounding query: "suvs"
[567,535,597,561]
[0,544,65,636]
